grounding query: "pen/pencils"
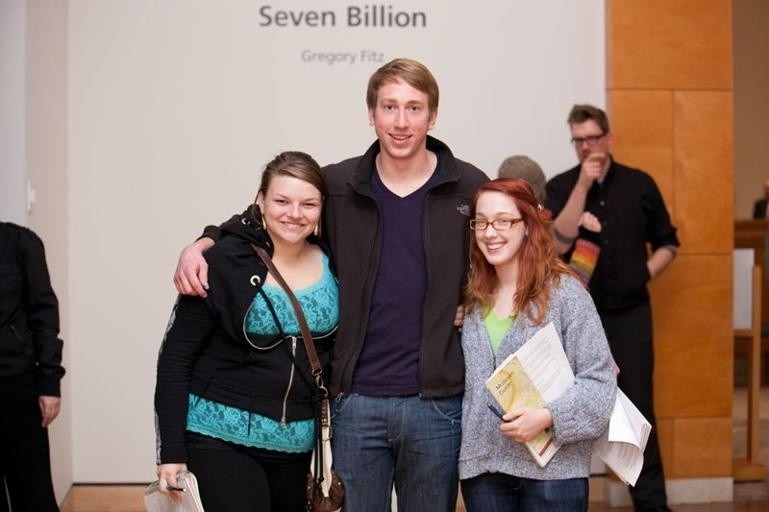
[167,486,186,493]
[487,402,511,423]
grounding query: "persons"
[170,57,495,511]
[456,178,623,510]
[1,222,66,511]
[153,152,472,512]
[499,154,604,288]
[542,102,683,511]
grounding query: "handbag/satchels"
[307,471,345,512]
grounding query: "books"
[144,471,203,511]
[486,320,579,470]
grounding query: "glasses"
[571,133,605,146]
[470,219,523,230]
